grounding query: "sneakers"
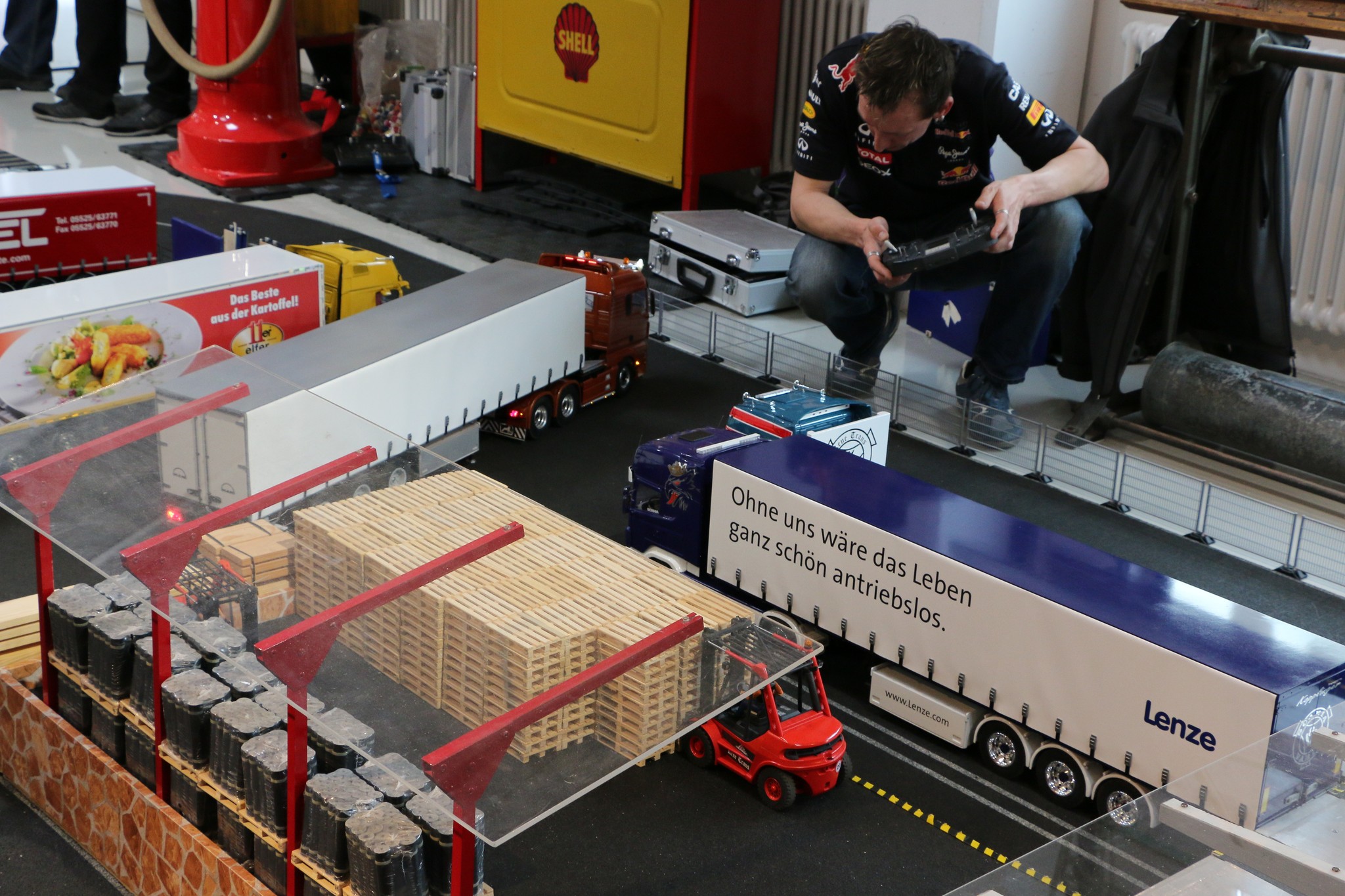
[103,99,191,136]
[955,359,1022,446]
[33,94,114,127]
[830,345,881,394]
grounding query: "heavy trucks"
[619,422,1345,850]
[0,238,412,515]
[152,247,657,525]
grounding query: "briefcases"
[647,209,806,316]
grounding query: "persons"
[784,12,1109,447]
[0,0,367,137]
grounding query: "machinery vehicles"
[677,615,853,815]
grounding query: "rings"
[995,209,1008,215]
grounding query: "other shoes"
[0,64,52,90]
